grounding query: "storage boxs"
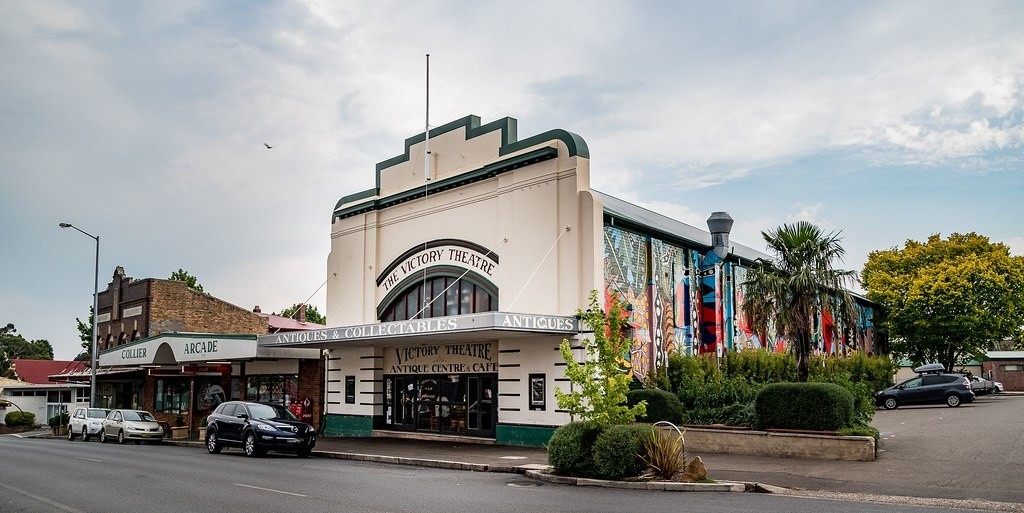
[914,363,945,375]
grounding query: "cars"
[99,409,164,445]
[963,375,1003,395]
[875,373,975,409]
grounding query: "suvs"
[67,407,114,441]
[205,401,316,456]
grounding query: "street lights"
[59,221,100,408]
